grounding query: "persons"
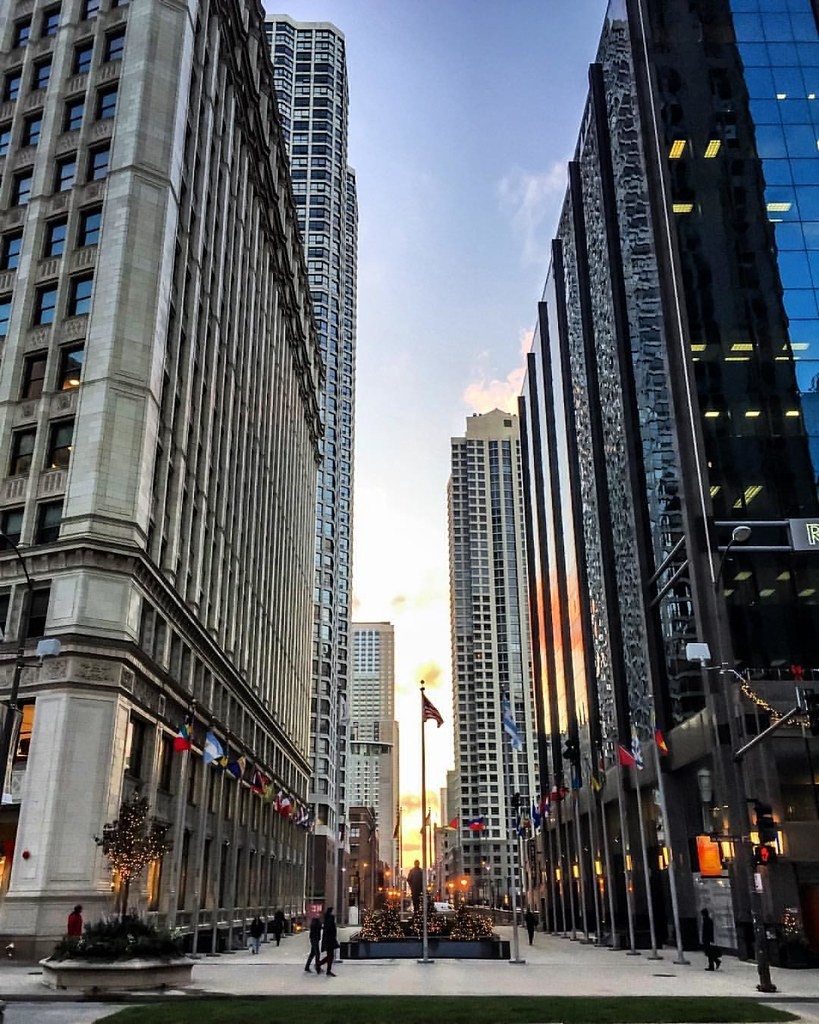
[407,860,422,911]
[251,914,263,954]
[274,906,286,946]
[303,907,339,977]
[700,908,722,971]
[68,905,83,941]
[525,908,537,945]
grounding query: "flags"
[423,695,443,728]
[449,818,458,829]
[515,752,606,835]
[654,725,668,755]
[503,694,523,751]
[619,745,635,766]
[469,818,483,830]
[203,732,223,763]
[219,743,314,832]
[174,723,190,751]
[631,734,645,771]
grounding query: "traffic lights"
[803,687,819,735]
[754,845,776,864]
[754,801,774,842]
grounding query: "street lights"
[712,525,777,993]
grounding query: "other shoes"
[305,968,312,972]
[326,972,336,977]
[314,965,322,975]
